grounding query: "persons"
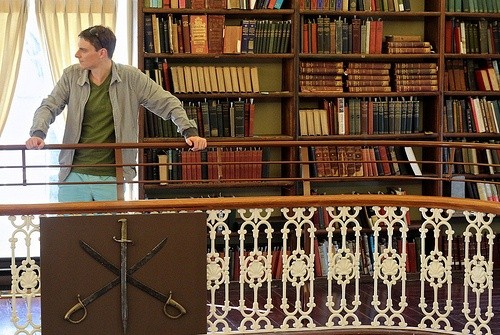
[24,25,207,203]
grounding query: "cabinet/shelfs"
[138,0,500,290]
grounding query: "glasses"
[89,27,105,48]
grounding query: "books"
[140,0,499,276]
